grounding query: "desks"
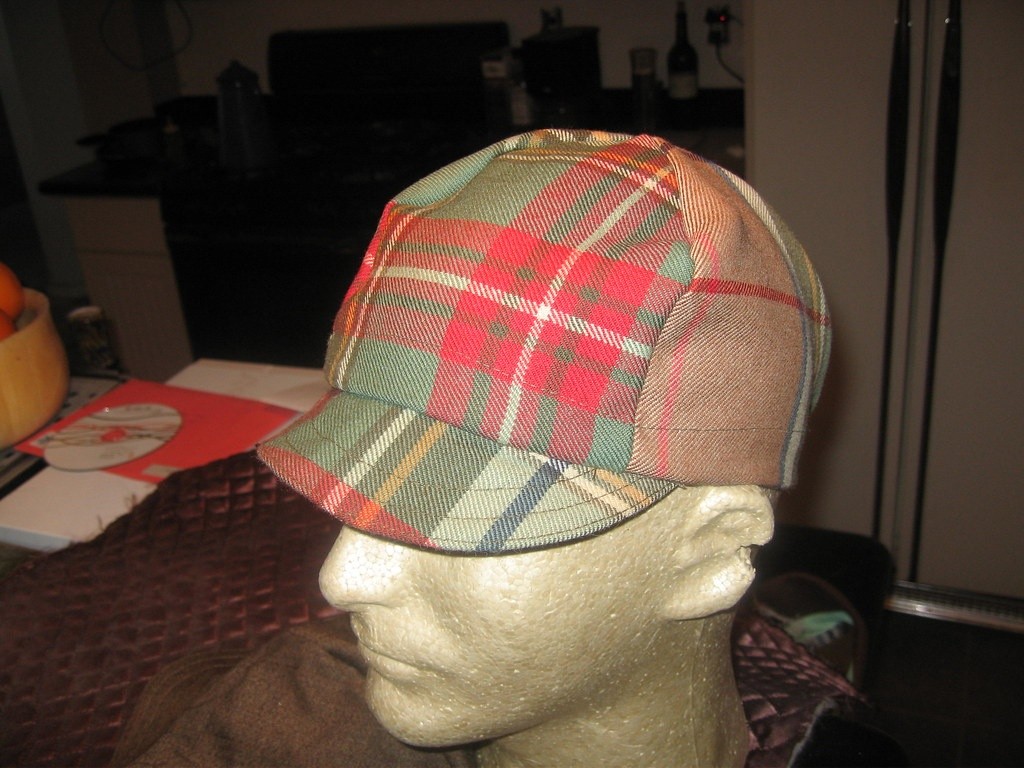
[0,359,897,767]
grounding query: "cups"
[632,48,656,91]
[1,289,70,448]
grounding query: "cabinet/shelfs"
[37,189,185,375]
[741,0,1024,603]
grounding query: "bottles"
[666,1,698,106]
[506,47,539,133]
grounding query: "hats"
[254,127,832,553]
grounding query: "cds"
[43,405,182,471]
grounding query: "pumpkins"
[0,260,24,340]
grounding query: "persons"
[132,132,831,768]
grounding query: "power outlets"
[707,4,732,45]
